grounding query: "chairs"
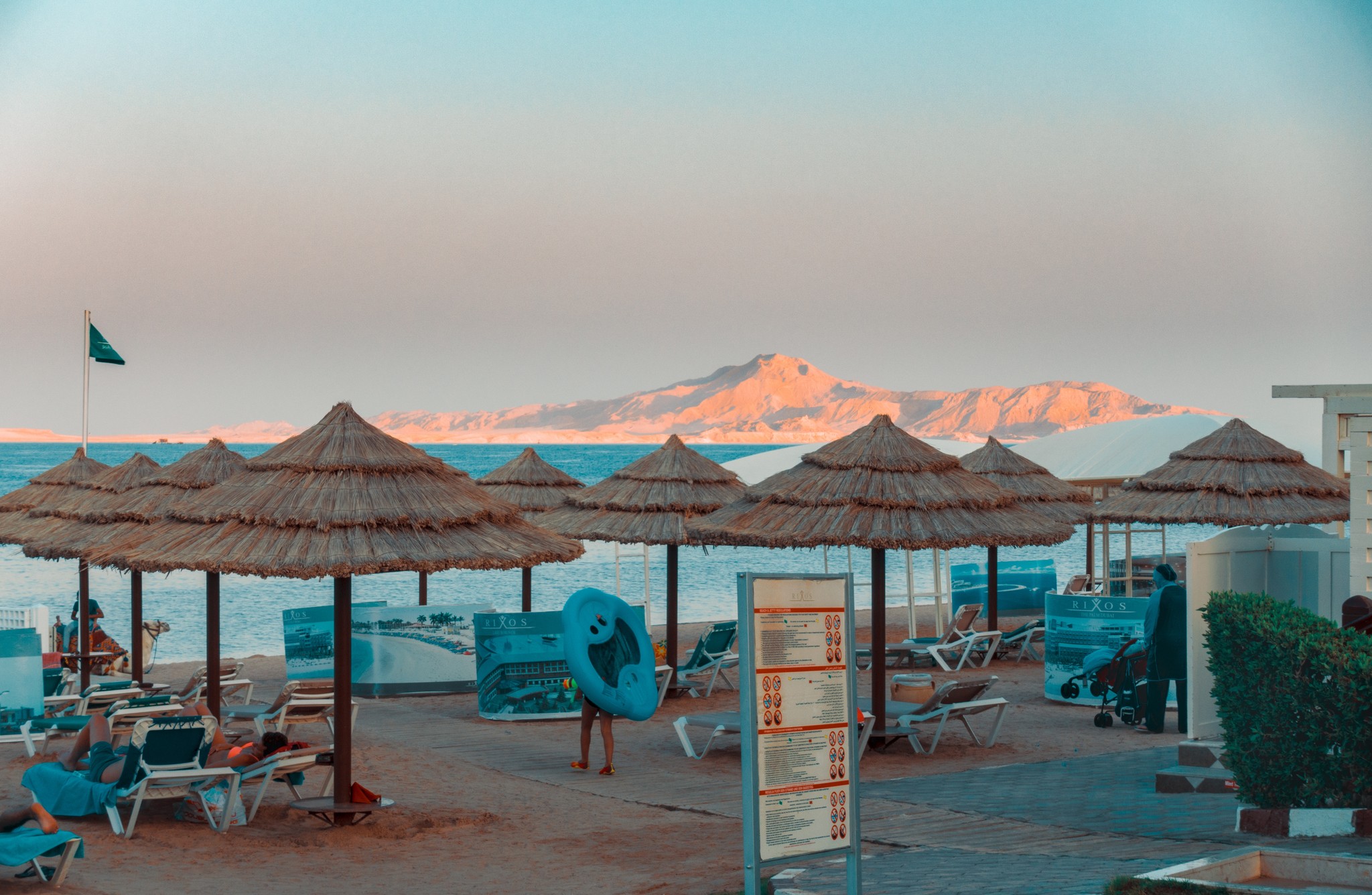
[654,574,1091,761]
[0,669,359,888]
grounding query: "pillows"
[652,639,667,666]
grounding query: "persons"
[55,714,126,784]
[1134,563,1187,734]
[0,803,59,834]
[71,591,104,631]
[174,700,290,769]
[570,618,618,775]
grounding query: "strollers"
[1332,595,1372,639]
[1059,638,1148,728]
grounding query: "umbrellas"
[474,435,750,699]
[1091,417,1354,528]
[0,402,583,828]
[685,411,1090,756]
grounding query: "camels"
[53,620,170,675]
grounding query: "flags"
[89,323,125,365]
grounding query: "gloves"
[563,679,569,689]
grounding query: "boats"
[151,438,184,444]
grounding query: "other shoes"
[599,762,615,775]
[1134,723,1149,731]
[570,760,590,770]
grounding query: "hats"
[1337,595,1372,637]
